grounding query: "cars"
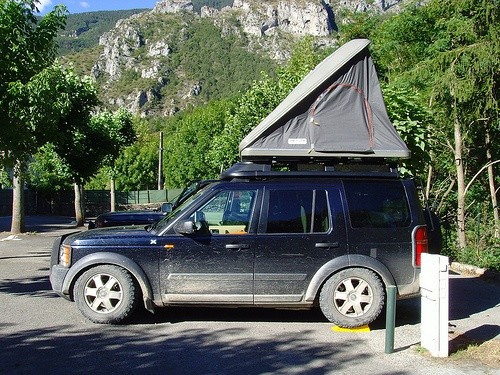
[47,159,442,326]
